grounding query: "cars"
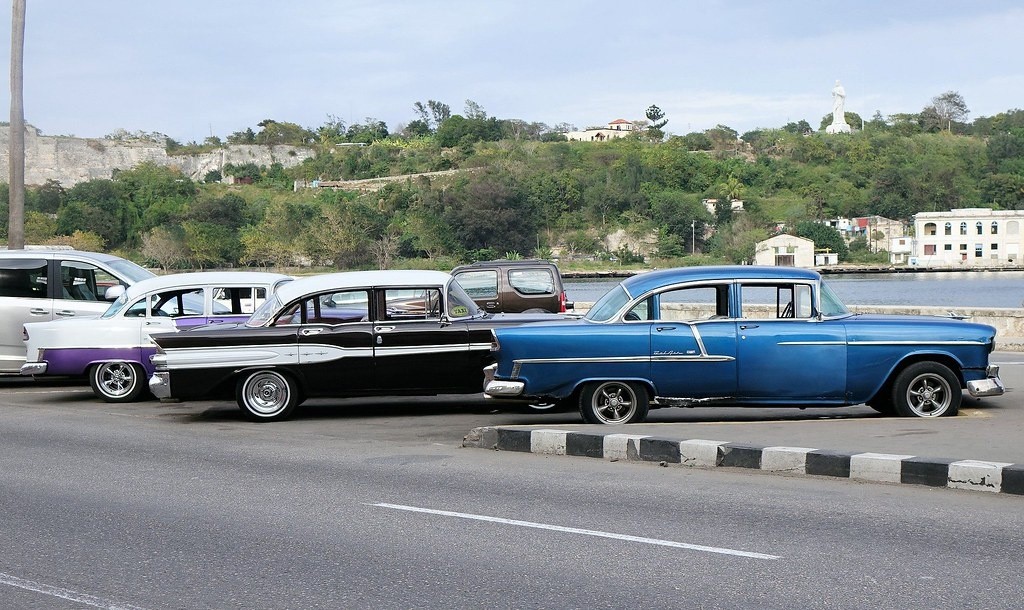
[479,266,1005,428]
[19,270,410,403]
[0,247,228,376]
[148,270,578,424]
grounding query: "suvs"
[386,261,574,325]
